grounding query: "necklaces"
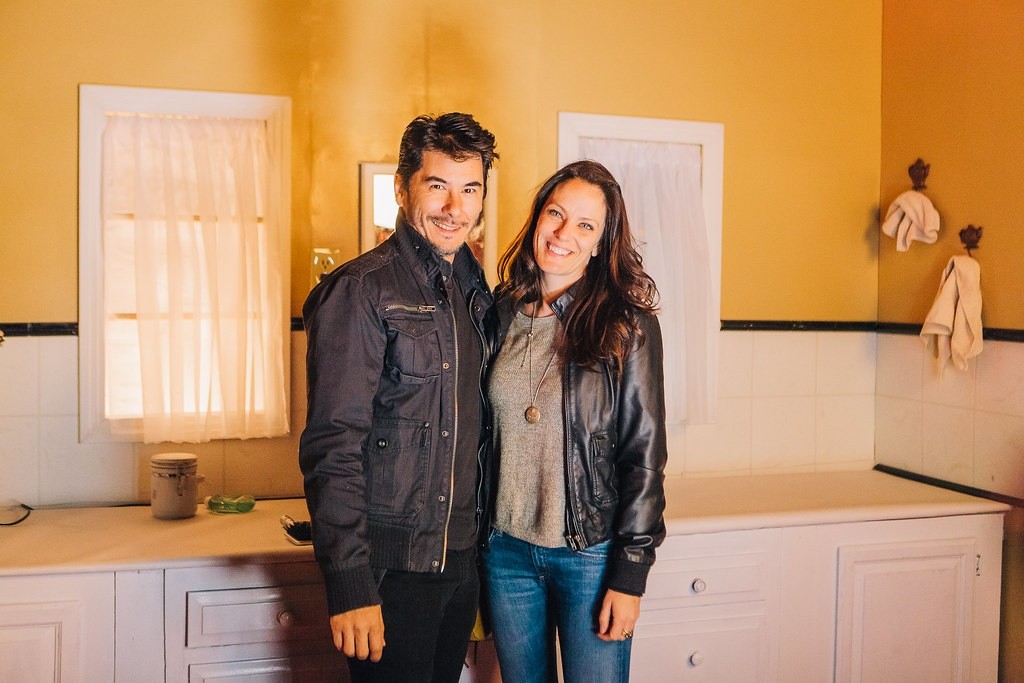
[525,298,564,426]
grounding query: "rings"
[622,629,633,639]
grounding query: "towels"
[916,255,986,380]
[881,188,942,253]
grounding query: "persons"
[298,113,499,683]
[477,160,670,682]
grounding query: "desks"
[0,469,1013,683]
